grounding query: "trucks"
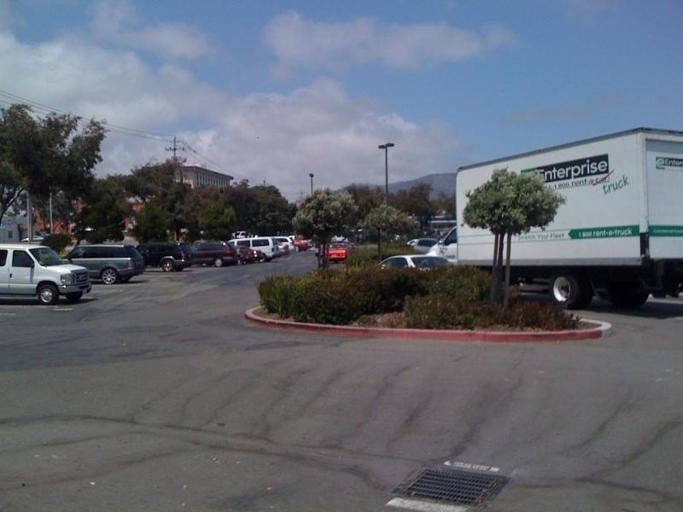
[430,128,683,312]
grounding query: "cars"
[350,227,450,276]
[324,241,352,260]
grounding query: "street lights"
[378,141,393,196]
[306,172,313,194]
